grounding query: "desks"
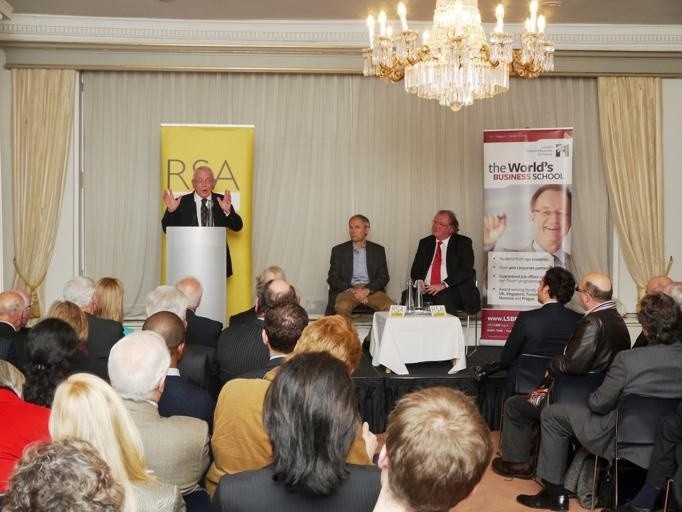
[369,311,467,376]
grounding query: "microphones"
[204,198,214,225]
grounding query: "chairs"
[446,269,478,359]
[496,352,682,512]
[327,283,378,317]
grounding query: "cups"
[424,301,433,314]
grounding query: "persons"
[490,267,682,512]
[482,184,573,308]
[160,166,244,280]
[1,268,494,512]
[324,214,399,319]
[401,209,482,318]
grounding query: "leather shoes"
[492,456,535,478]
[516,490,570,511]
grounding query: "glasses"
[532,209,569,217]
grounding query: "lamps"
[361,0,555,112]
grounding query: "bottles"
[405,279,414,313]
[413,280,424,312]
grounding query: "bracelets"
[440,282,446,288]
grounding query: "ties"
[431,241,443,285]
[200,198,208,226]
